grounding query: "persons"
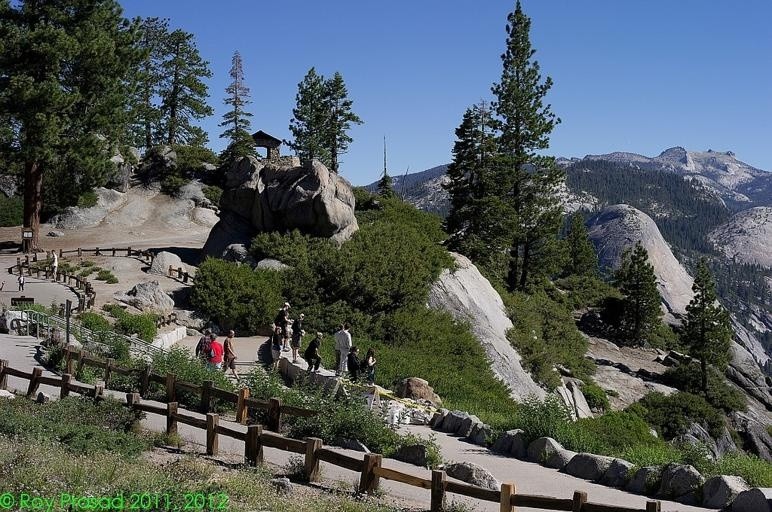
[223,327,240,382]
[18,274,25,291]
[195,328,213,367]
[50,249,59,283]
[204,332,225,371]
[270,302,378,388]
